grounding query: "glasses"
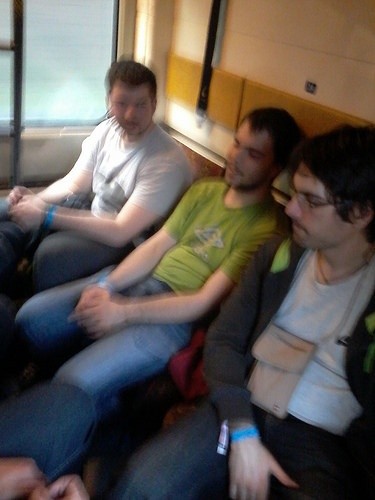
[289,174,334,209]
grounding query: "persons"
[17,106,304,422]
[0,382,98,500]
[116,123,375,500]
[1,59,194,289]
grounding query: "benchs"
[160,123,290,211]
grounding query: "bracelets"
[99,276,115,295]
[230,426,261,441]
[41,204,59,229]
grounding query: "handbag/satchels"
[169,330,209,399]
[247,323,317,419]
